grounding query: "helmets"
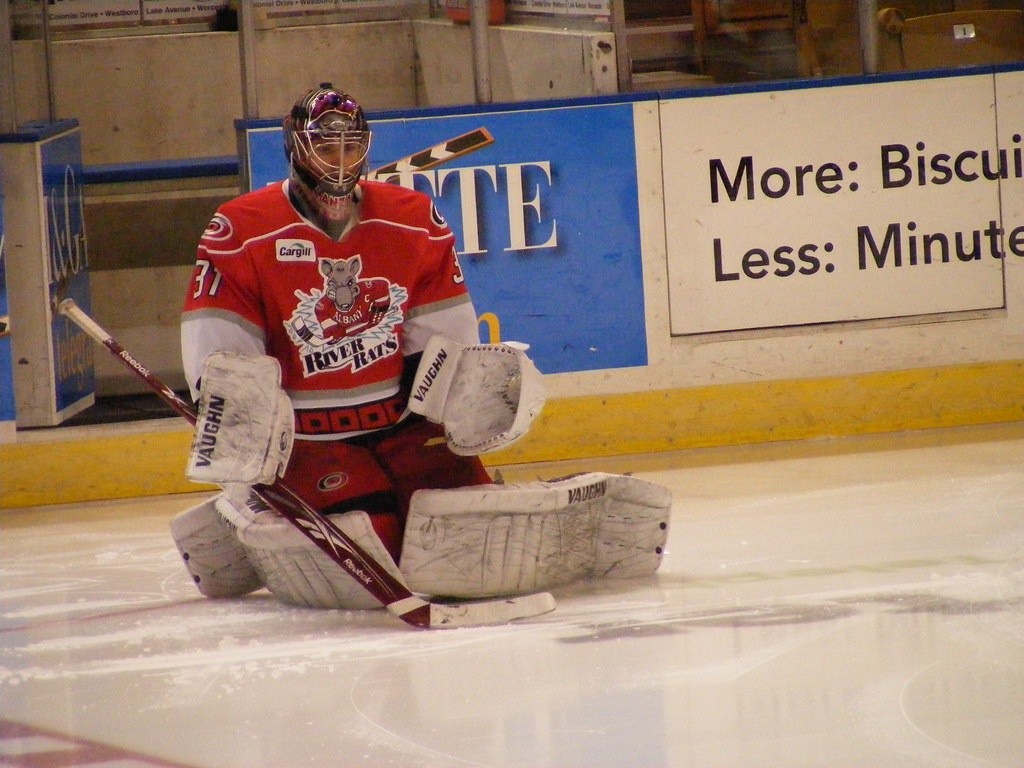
[281,80,373,220]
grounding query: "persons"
[164,82,672,609]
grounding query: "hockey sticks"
[55,297,559,629]
